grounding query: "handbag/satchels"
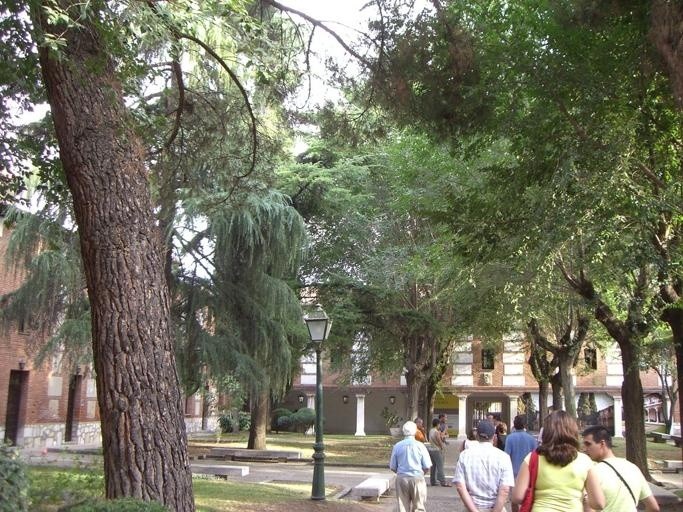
[518,489,535,511]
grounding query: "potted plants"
[385,414,404,436]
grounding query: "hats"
[476,419,496,437]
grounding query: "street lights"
[304,303,333,500]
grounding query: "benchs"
[650,431,683,474]
[211,447,300,463]
[353,473,393,502]
[190,464,250,481]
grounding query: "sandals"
[431,480,453,488]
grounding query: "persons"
[511,410,606,512]
[461,412,507,452]
[452,419,515,512]
[504,415,543,512]
[582,426,660,512]
[414,412,453,487]
[390,420,433,512]
